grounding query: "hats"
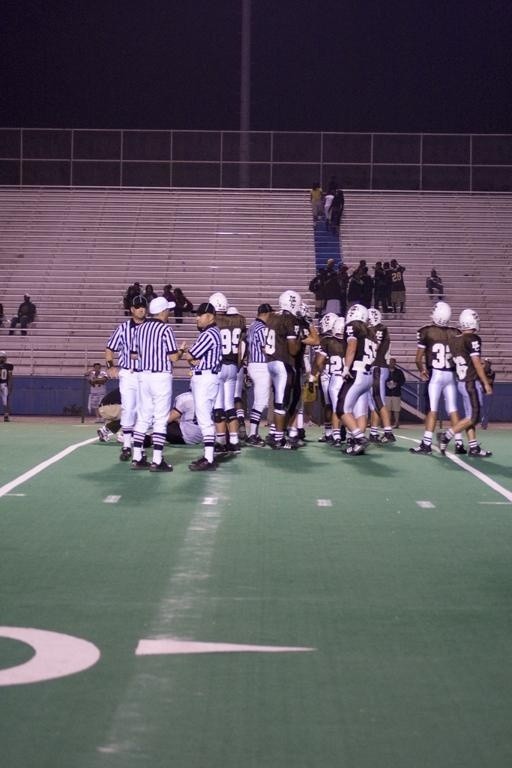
[132,295,149,309]
[150,296,176,314]
[228,303,277,313]
[191,302,217,316]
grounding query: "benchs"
[337,188,512,382]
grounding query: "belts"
[195,370,218,375]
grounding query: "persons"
[8,292,35,335]
[410,300,469,455]
[124,282,193,327]
[426,269,444,300]
[436,309,493,457]
[0,303,3,328]
[0,352,13,422]
[311,176,345,233]
[84,296,406,475]
[309,258,406,319]
[480,358,496,430]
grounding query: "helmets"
[209,292,229,312]
[0,351,7,360]
[279,290,479,339]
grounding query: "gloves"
[342,366,354,382]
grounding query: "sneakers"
[150,457,173,471]
[469,445,493,458]
[98,425,111,442]
[189,427,396,470]
[119,446,133,460]
[410,443,433,455]
[455,444,468,454]
[436,432,451,456]
[131,456,152,470]
